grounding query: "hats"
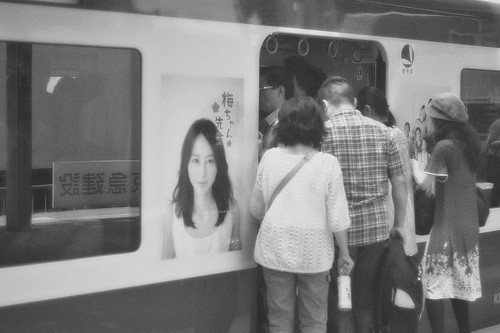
[425,94,468,122]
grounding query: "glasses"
[259,86,274,95]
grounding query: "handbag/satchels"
[414,188,435,235]
[476,186,489,226]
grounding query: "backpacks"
[372,238,423,333]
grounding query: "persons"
[356,85,418,333]
[258,65,294,163]
[318,76,409,333]
[416,93,482,333]
[162,119,242,260]
[486,119,500,207]
[404,99,434,168]
[250,95,355,333]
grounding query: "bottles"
[337,267,352,310]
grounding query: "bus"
[0,0,500,333]
